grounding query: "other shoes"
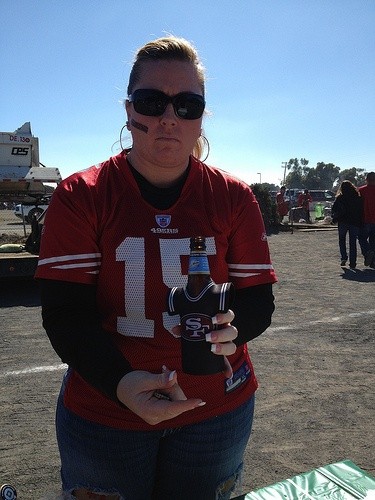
[340,259,346,266]
[349,264,356,269]
[370,259,374,268]
[364,251,374,266]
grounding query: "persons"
[38,36,276,500]
[331,180,362,268]
[357,171,375,267]
[276,186,289,230]
[298,189,312,224]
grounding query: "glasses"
[126,88,206,121]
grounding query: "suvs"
[285,189,299,201]
[302,189,336,210]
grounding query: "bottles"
[182,235,224,376]
